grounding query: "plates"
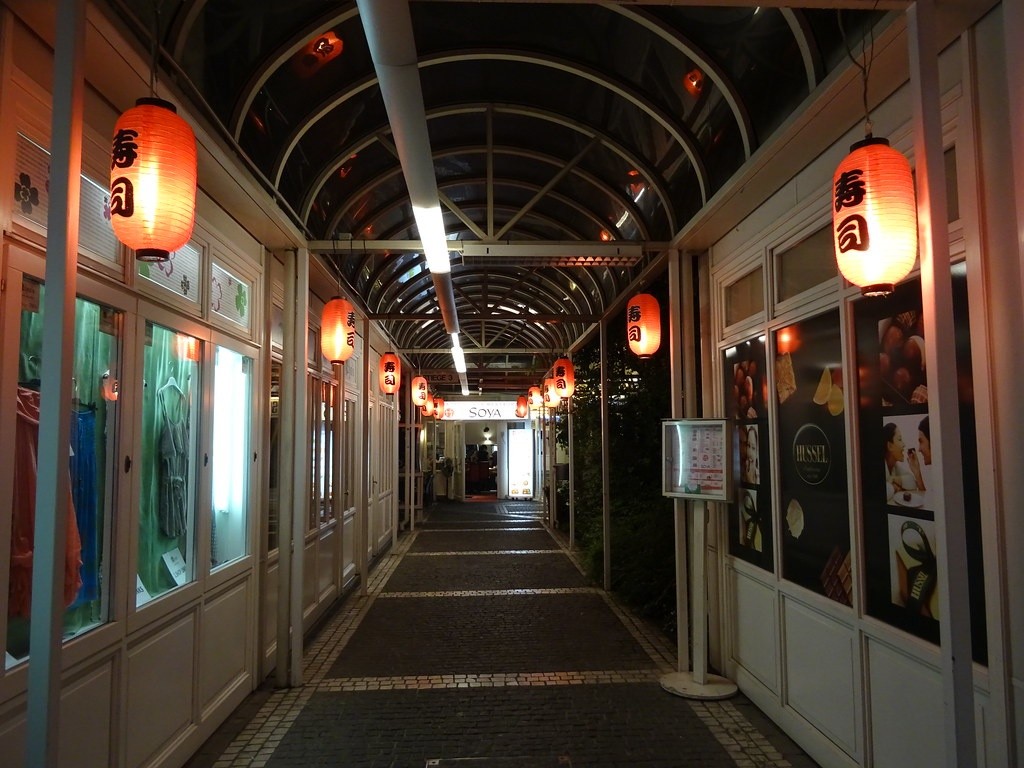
[894,492,924,507]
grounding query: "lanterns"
[626,293,662,361]
[412,374,445,421]
[320,297,356,368]
[377,352,402,402]
[683,69,704,101]
[295,31,344,79]
[106,93,199,261]
[832,133,917,297]
[516,356,575,419]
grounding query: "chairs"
[469,461,490,492]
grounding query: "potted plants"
[544,481,570,528]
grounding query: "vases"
[553,463,570,480]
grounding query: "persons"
[738,425,759,485]
[469,444,499,495]
[883,414,932,513]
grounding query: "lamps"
[355,0,417,63]
[412,205,450,274]
[372,62,439,209]
[424,275,471,396]
